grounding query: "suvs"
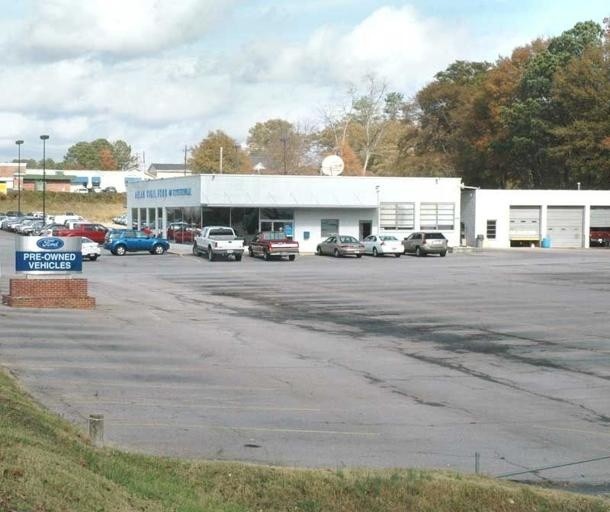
[402,232,449,257]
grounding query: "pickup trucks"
[248,231,300,262]
[192,225,245,261]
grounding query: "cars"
[75,185,118,193]
[132,217,202,243]
[360,235,403,257]
[0,209,169,260]
[316,234,364,258]
[590,227,610,247]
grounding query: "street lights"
[40,134,49,227]
[278,137,286,175]
[15,140,23,211]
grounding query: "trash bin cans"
[477,235,483,248]
